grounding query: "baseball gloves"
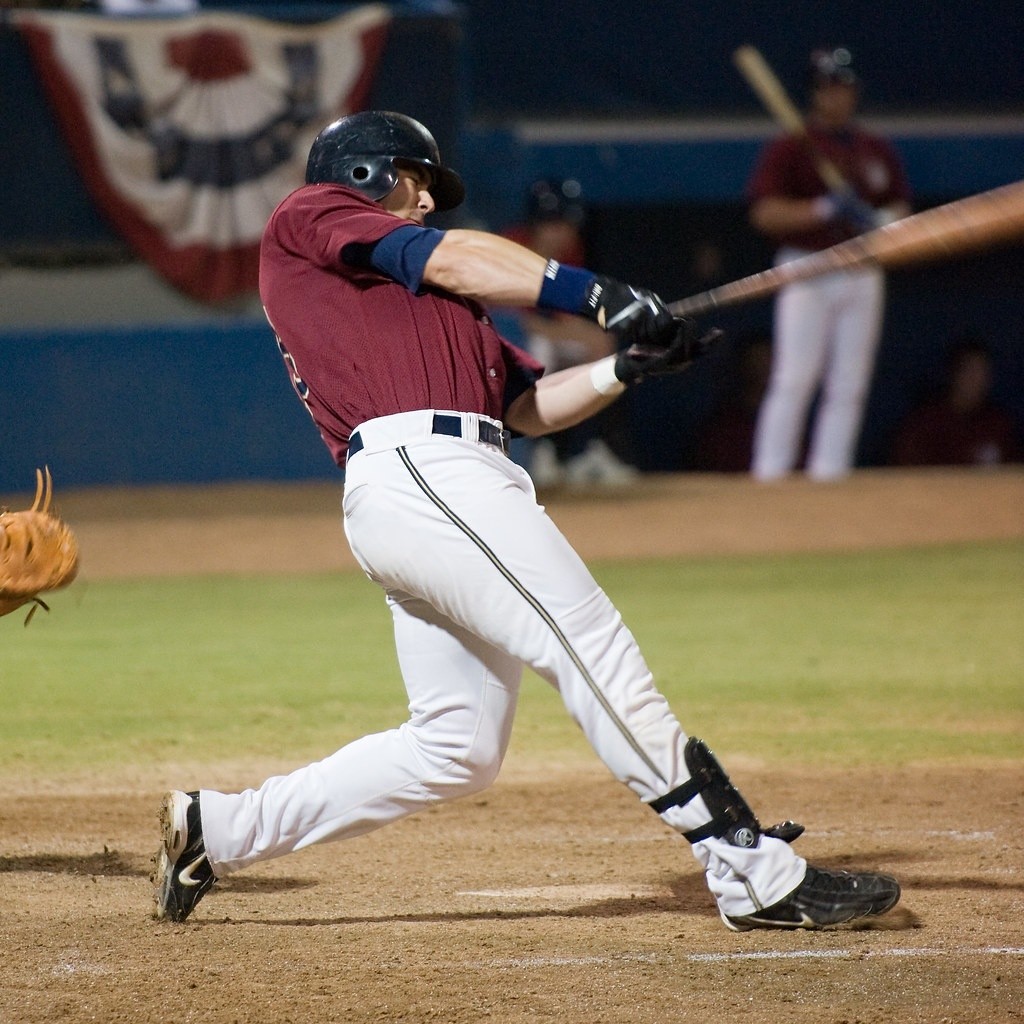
[1,511,79,615]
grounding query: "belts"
[349,415,500,454]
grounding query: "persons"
[153,108,901,934]
[749,51,910,486]
[501,181,636,489]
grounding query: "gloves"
[581,276,723,388]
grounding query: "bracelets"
[536,262,598,311]
[591,355,628,396]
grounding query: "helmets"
[305,111,467,214]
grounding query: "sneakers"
[721,863,901,933]
[150,788,215,921]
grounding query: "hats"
[524,180,587,227]
[806,49,877,96]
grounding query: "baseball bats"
[736,44,858,203]
[664,183,1024,309]
